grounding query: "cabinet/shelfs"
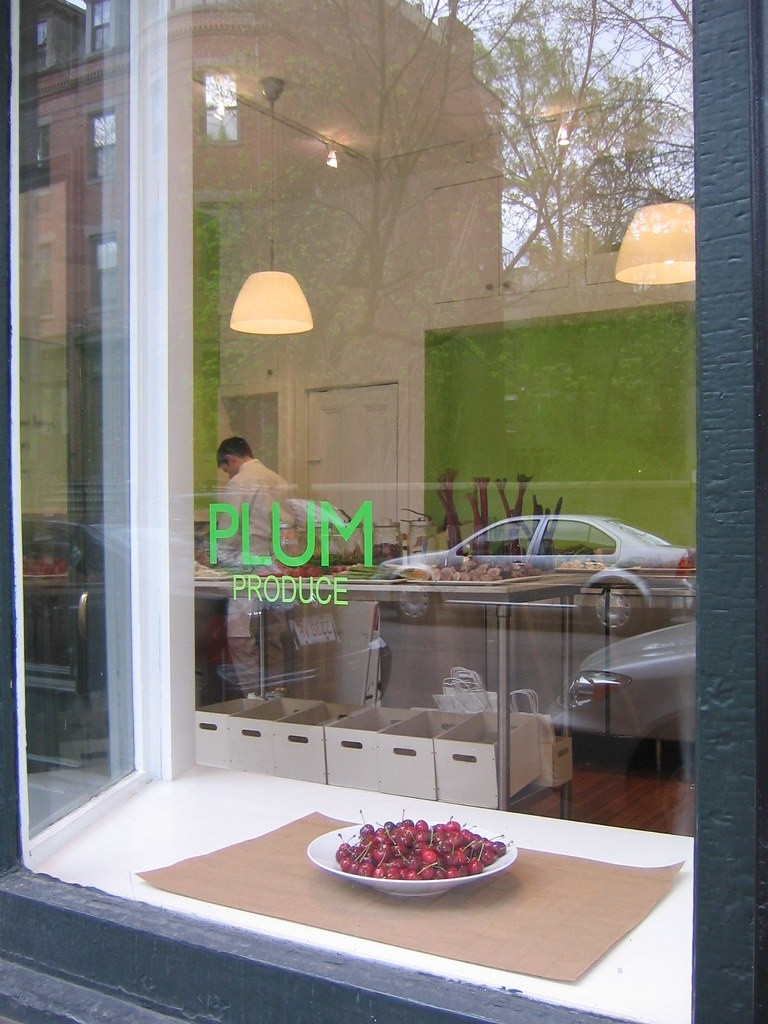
[377,587,698,772]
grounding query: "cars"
[21,513,345,727]
[549,621,695,786]
[373,514,696,637]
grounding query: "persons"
[214,437,298,700]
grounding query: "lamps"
[229,77,314,336]
[614,200,696,286]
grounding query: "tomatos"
[258,559,324,578]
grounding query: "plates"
[307,821,518,897]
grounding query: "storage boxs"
[25,654,108,769]
[194,697,574,810]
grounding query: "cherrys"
[337,809,513,880]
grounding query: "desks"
[25,570,628,826]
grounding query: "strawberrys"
[23,555,69,574]
